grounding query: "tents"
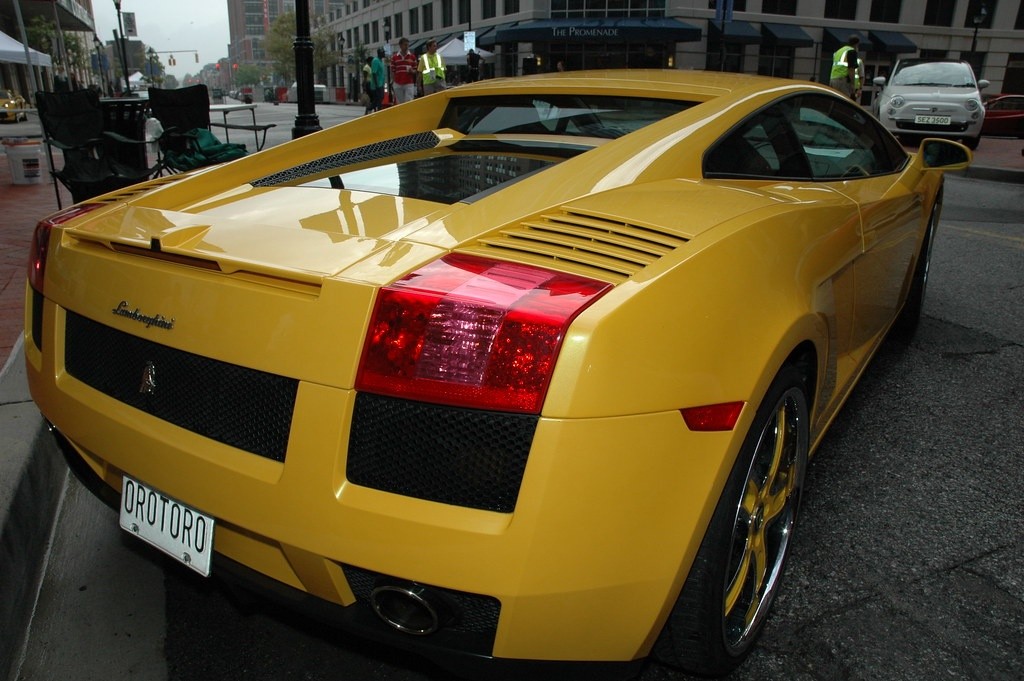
[0,31,54,110]
[418,38,495,85]
[123,72,156,91]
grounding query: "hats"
[377,47,385,53]
[366,56,374,63]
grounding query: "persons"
[363,47,386,115]
[830,35,866,119]
[108,81,114,97]
[557,59,564,72]
[417,40,447,96]
[390,38,418,104]
[466,49,485,84]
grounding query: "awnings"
[761,23,814,48]
[708,18,763,44]
[868,31,917,53]
[407,21,518,52]
[496,18,702,41]
[823,28,872,48]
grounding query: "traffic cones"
[382,82,393,108]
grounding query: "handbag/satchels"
[380,92,390,105]
[360,92,370,105]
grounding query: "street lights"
[114,0,130,95]
[339,36,346,87]
[383,21,392,102]
[93,34,107,98]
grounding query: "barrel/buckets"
[4,144,41,185]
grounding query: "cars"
[874,56,991,147]
[228,90,245,101]
[980,93,1024,137]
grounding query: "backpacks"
[466,53,480,68]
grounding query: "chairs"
[474,102,549,134]
[714,136,771,173]
[34,82,277,203]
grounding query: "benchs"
[211,122,277,151]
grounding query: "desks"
[209,104,259,151]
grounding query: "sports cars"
[20,64,972,680]
[0,90,27,123]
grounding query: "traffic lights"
[216,64,220,71]
[234,64,237,71]
